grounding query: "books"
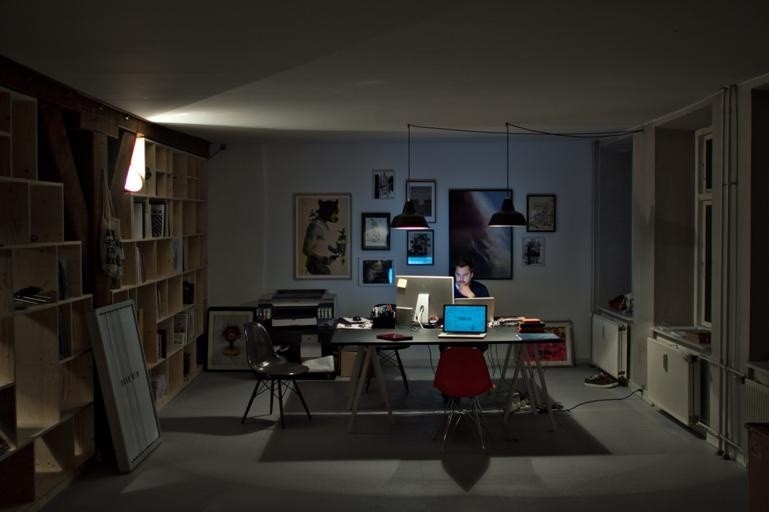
[10,255,93,362]
[132,194,209,403]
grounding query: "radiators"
[590,312,630,385]
[741,374,769,465]
[647,335,702,430]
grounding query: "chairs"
[364,303,411,393]
[433,345,498,454]
[241,320,313,429]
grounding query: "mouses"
[353,315,362,321]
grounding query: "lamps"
[391,124,430,230]
[488,119,526,228]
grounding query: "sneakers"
[589,371,621,384]
[583,373,616,388]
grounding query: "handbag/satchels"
[100,216,123,279]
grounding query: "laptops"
[455,296,496,324]
[438,304,488,339]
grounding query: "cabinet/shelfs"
[257,292,337,381]
[0,85,209,512]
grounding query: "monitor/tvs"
[395,275,455,325]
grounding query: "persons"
[302,196,342,276]
[438,259,492,407]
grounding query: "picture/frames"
[357,255,396,288]
[407,229,435,265]
[405,178,437,224]
[203,306,257,373]
[293,192,352,281]
[448,189,514,281]
[526,193,556,233]
[361,212,391,251]
[513,319,575,369]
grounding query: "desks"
[744,423,768,512]
[329,324,562,434]
